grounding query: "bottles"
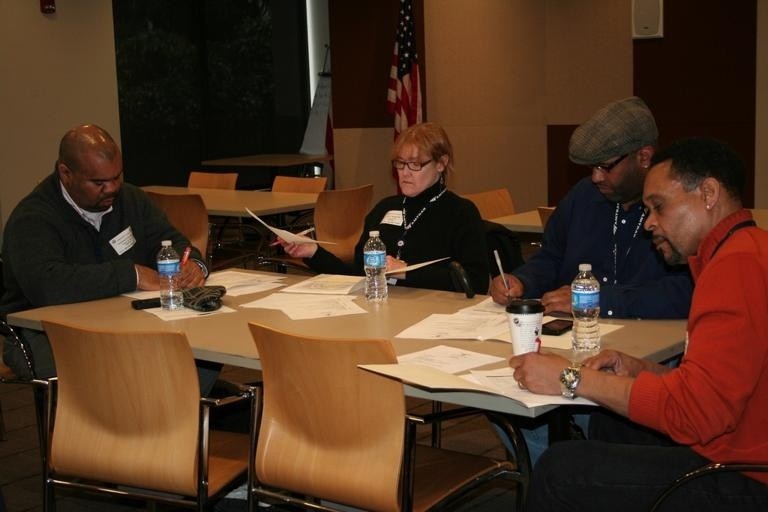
[156,241,184,311]
[363,231,388,303]
[571,263,600,353]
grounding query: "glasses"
[600,155,628,172]
[390,159,432,172]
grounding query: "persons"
[0,124,209,385]
[509,130,768,512]
[277,123,490,294]
[491,95,695,321]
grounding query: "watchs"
[559,361,581,401]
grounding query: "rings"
[517,380,526,391]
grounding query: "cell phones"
[131,296,180,310]
[542,319,573,336]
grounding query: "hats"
[568,96,657,168]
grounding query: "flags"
[386,0,424,196]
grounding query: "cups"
[504,298,545,356]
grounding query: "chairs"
[642,457,767,511]
[128,154,766,290]
[234,319,533,512]
[25,319,259,511]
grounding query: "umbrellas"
[448,259,518,466]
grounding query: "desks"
[0,268,697,512]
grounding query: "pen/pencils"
[534,337,541,353]
[180,246,192,265]
[494,249,512,305]
[268,226,316,248]
